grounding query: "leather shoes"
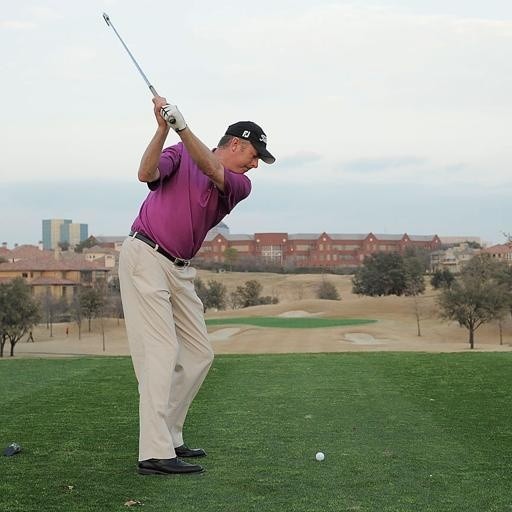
[174,444,207,458]
[136,457,204,476]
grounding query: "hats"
[224,120,277,164]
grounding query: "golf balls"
[315,452,324,461]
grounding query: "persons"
[118,95,277,474]
[27,330,34,342]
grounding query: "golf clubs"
[103,12,176,124]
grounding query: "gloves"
[160,103,188,133]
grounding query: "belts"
[129,231,190,269]
